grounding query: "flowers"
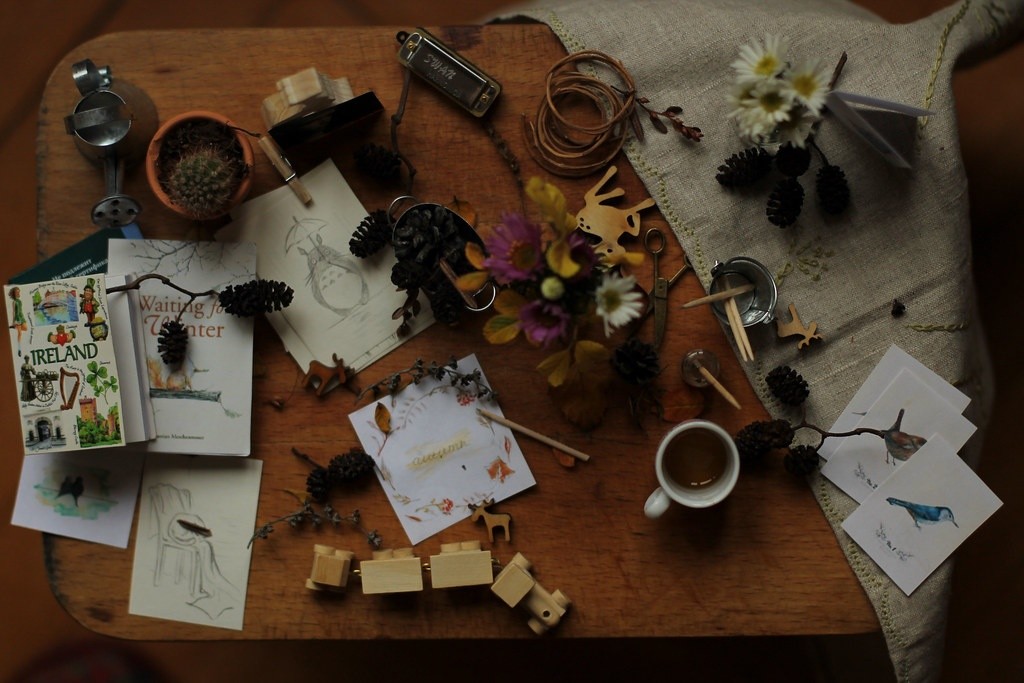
[454,175,649,390]
[723,28,833,150]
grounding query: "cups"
[644,420,740,519]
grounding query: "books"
[8,222,146,285]
[4,273,157,455]
[108,236,257,457]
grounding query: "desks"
[35,25,952,636]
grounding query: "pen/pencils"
[694,360,741,411]
[476,407,590,463]
[681,284,754,308]
[718,275,754,362]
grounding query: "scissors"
[625,228,693,349]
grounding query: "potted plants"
[146,111,255,221]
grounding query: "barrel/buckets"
[709,256,777,327]
